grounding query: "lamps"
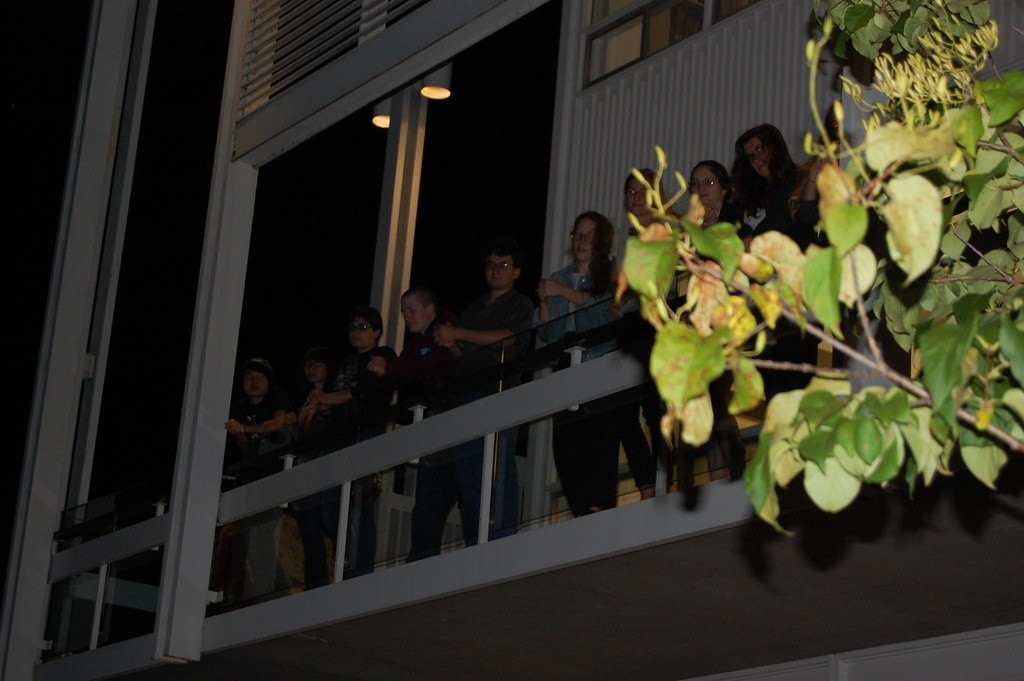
[420,60,453,100]
[372,97,391,128]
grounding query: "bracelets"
[241,425,244,431]
[807,178,818,185]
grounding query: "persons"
[224,103,839,591]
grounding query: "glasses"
[745,146,766,164]
[625,187,647,199]
[244,375,266,382]
[484,260,513,269]
[570,231,593,244]
[302,361,323,368]
[688,176,720,190]
[348,324,370,332]
